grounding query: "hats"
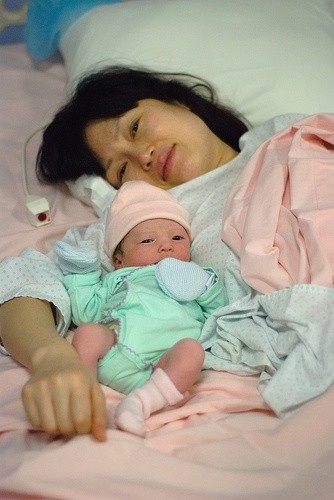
[104,179,193,257]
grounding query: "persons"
[65,179,230,437]
[1,58,333,443]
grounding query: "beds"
[0,41,332,500]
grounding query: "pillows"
[51,1,333,133]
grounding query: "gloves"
[50,239,102,275]
[152,258,208,304]
